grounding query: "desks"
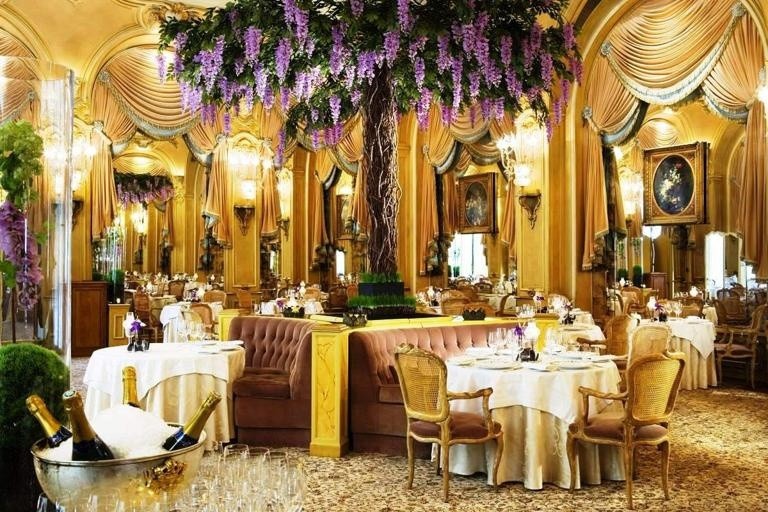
[86,336,246,443]
[637,315,718,390]
[432,347,631,488]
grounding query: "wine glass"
[31,444,305,512]
[545,314,601,362]
[176,319,206,350]
[672,299,683,324]
[488,327,519,361]
[126,312,134,350]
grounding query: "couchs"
[349,319,533,458]
[228,316,312,446]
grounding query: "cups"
[514,303,535,320]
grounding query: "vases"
[612,237,629,281]
[631,237,650,286]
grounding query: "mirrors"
[440,157,513,290]
[603,92,763,296]
[97,197,174,278]
[323,173,368,276]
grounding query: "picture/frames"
[458,175,498,233]
[643,143,707,229]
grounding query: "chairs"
[615,322,670,390]
[714,302,768,390]
[605,282,767,321]
[565,351,687,506]
[575,315,634,380]
[126,279,227,338]
[391,344,508,498]
[228,278,351,315]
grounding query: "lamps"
[132,211,148,264]
[221,140,270,198]
[333,191,357,241]
[496,122,547,183]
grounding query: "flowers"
[0,122,52,342]
[109,169,174,211]
[144,1,587,171]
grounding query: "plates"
[216,340,244,351]
[476,362,513,370]
[551,359,591,370]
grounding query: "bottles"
[162,389,223,451]
[25,393,73,448]
[121,366,142,408]
[62,390,115,460]
